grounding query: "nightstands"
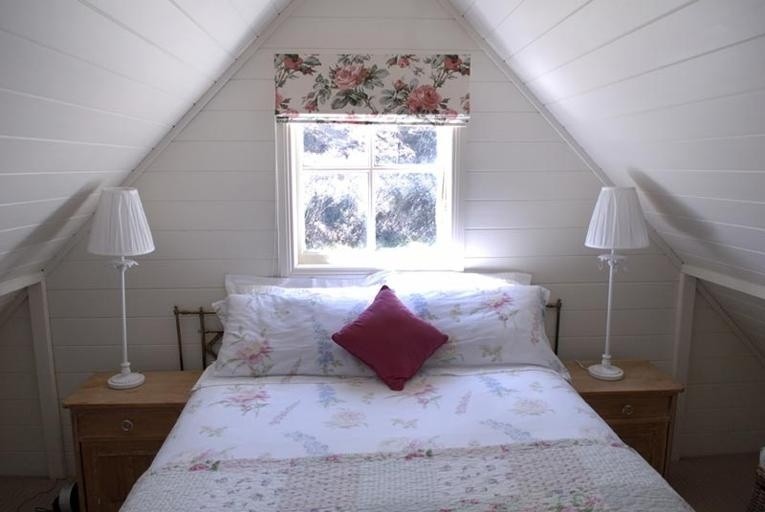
[60,370,198,510]
[564,358,685,474]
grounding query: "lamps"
[85,185,156,391]
[585,186,650,384]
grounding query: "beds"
[122,298,697,512]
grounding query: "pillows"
[330,285,449,391]
[210,285,573,381]
